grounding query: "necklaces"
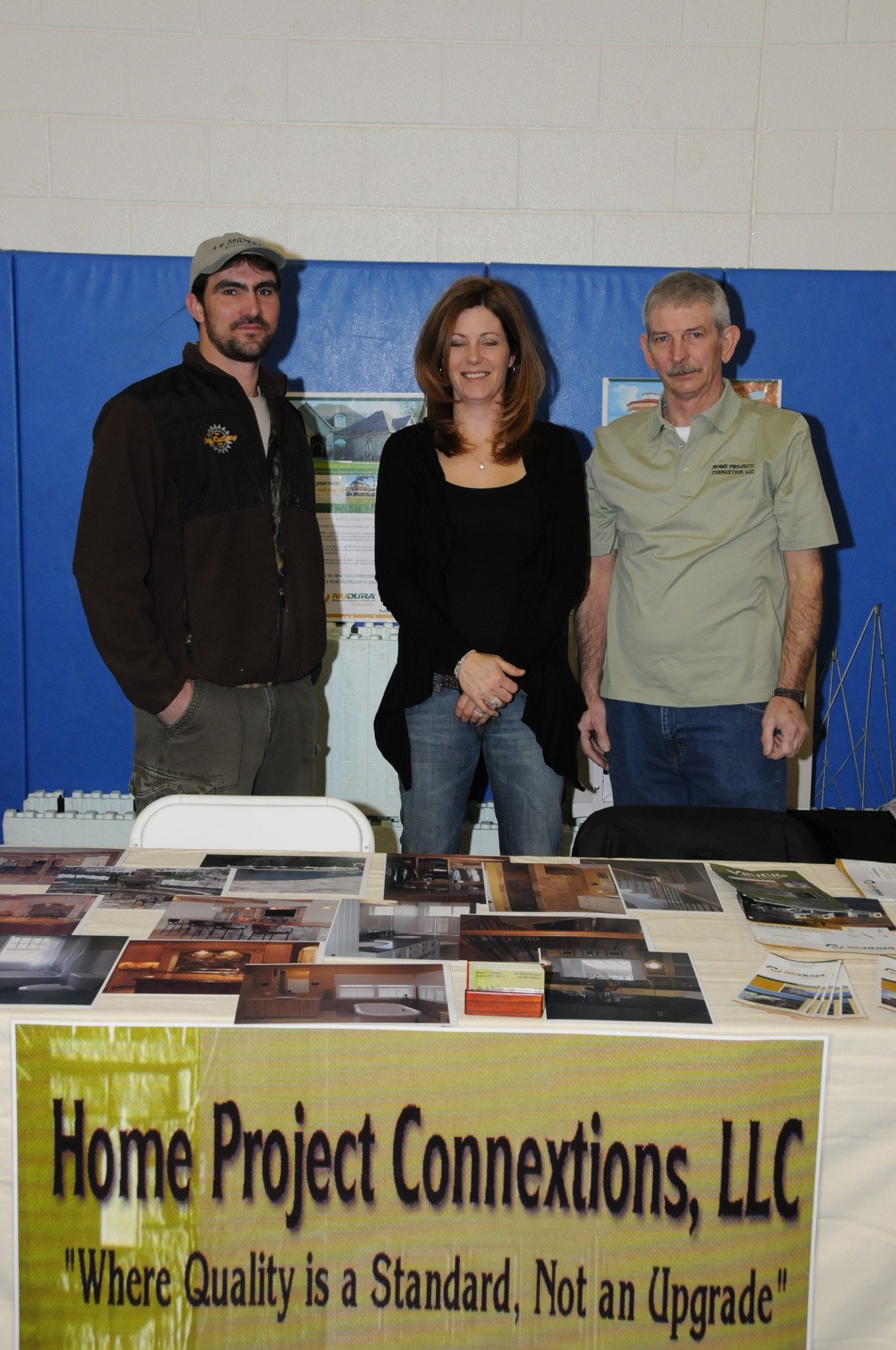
[464,444,492,469]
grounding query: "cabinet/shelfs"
[577,896,626,914]
[638,951,697,978]
[358,937,440,959]
[243,1001,321,1018]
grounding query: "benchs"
[159,918,293,941]
[134,899,157,906]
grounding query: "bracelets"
[454,650,476,679]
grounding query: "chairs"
[571,804,896,865]
[128,793,376,853]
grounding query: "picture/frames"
[559,950,623,957]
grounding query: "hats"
[189,232,285,291]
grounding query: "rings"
[488,696,498,708]
[474,709,485,718]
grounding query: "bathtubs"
[353,1003,421,1023]
[0,970,61,989]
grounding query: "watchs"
[773,687,807,710]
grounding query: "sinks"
[390,936,422,941]
[359,940,397,947]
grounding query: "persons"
[573,271,840,813]
[374,278,590,853]
[74,232,326,813]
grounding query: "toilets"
[18,972,102,1004]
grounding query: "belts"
[432,672,460,690]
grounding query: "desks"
[0,847,896,1350]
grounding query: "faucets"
[22,914,30,921]
[384,931,389,936]
